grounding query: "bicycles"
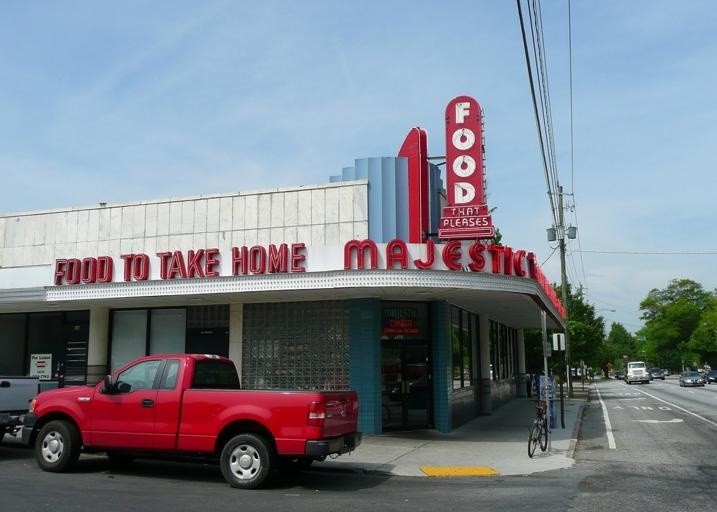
[528,399,549,458]
[382,404,391,425]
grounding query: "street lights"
[593,309,616,316]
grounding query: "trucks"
[624,362,650,385]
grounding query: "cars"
[679,369,717,387]
[614,371,626,380]
[389,364,429,401]
[650,367,670,381]
